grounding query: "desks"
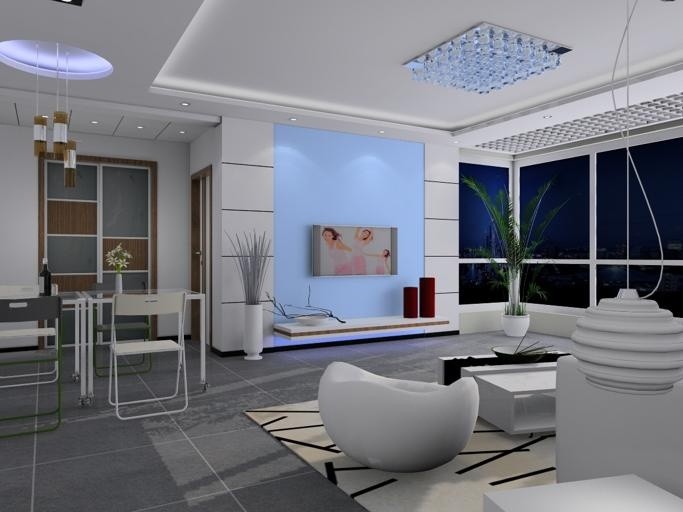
[81,288,208,407]
[482,474,683,512]
[0,290,87,408]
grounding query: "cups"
[48,283,58,297]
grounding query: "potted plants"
[222,228,274,361]
[458,172,576,337]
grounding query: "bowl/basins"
[492,346,548,363]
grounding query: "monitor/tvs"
[320,224,391,277]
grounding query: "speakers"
[391,226,398,275]
[312,224,320,276]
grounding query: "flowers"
[103,242,133,273]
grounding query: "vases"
[114,273,122,294]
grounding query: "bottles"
[38,258,51,298]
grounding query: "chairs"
[0,295,62,439]
[107,291,188,421]
[317,360,481,474]
[0,283,59,389]
[88,278,152,378]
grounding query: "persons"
[366,248,392,276]
[346,227,374,275]
[321,227,353,275]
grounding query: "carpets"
[242,398,557,512]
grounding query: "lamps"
[401,21,573,96]
[33,42,77,188]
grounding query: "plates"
[294,314,330,326]
